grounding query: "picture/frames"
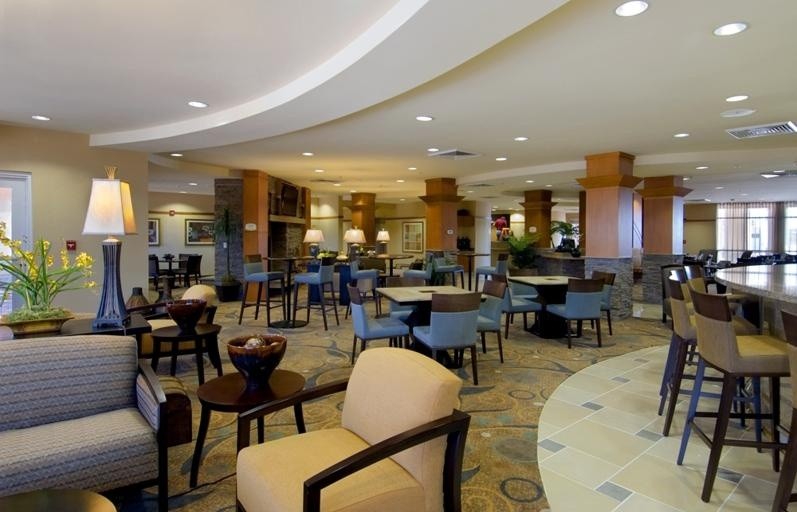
[402,221,424,253]
[148,216,159,246]
[183,219,214,246]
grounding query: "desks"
[372,285,488,369]
[450,252,489,291]
[189,369,307,492]
[150,325,223,380]
[507,275,582,339]
[371,255,414,278]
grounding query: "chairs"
[126,286,218,359]
[658,249,797,509]
[411,292,481,384]
[545,278,605,350]
[591,270,615,337]
[235,345,469,511]
[346,283,409,365]
[433,255,464,289]
[238,254,435,332]
[149,253,203,290]
[474,254,509,292]
[386,276,425,346]
[498,268,538,332]
[458,279,506,366]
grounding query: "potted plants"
[216,204,242,301]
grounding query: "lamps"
[79,165,137,330]
[378,229,390,255]
[344,226,367,258]
[303,228,324,255]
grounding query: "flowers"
[0,229,95,321]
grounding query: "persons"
[496,216,506,235]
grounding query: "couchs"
[0,334,178,512]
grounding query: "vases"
[0,306,78,338]
[226,334,288,389]
[169,300,206,331]
